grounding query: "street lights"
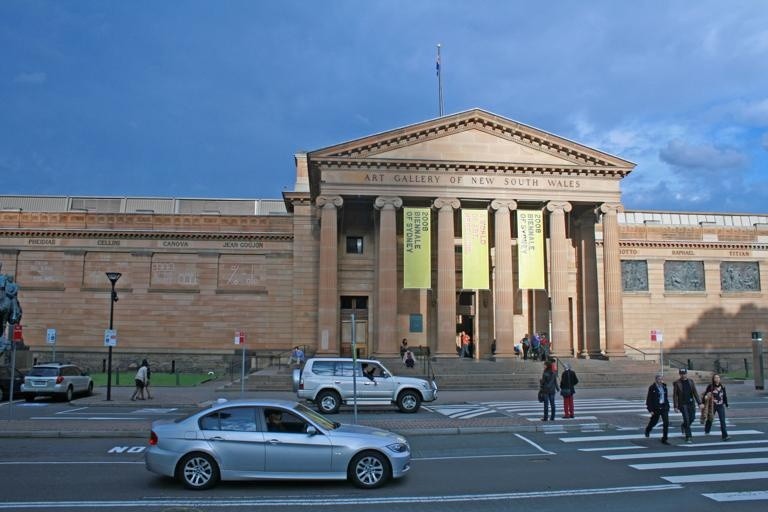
[104,272,121,401]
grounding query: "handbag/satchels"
[561,389,571,396]
[538,389,544,402]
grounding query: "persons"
[703,373,731,441]
[287,346,305,368]
[5,274,19,319]
[540,361,559,420]
[137,360,154,398]
[562,361,578,418]
[400,330,551,367]
[645,375,671,445]
[363,364,369,375]
[270,411,285,429]
[673,368,703,443]
[131,364,147,400]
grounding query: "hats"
[679,368,687,373]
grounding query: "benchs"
[409,345,429,358]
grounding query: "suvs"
[292,358,438,414]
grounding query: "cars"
[0,367,23,401]
[20,361,94,403]
[145,397,412,491]
